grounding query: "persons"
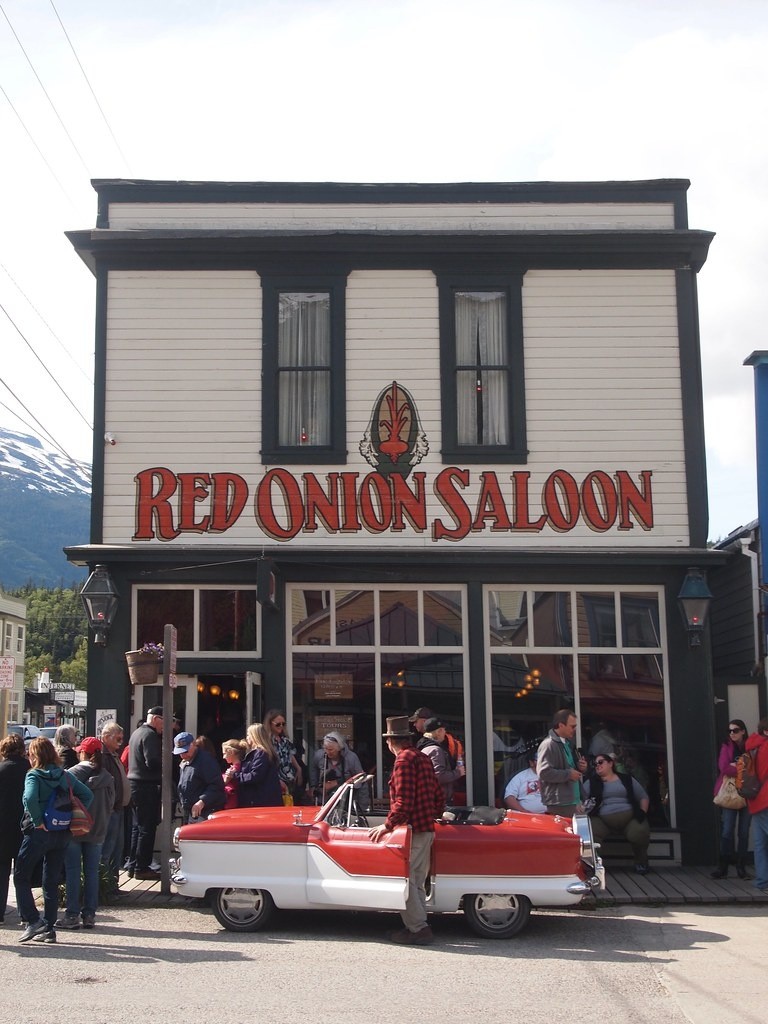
[745,720,768,896]
[711,720,754,881]
[0,707,650,946]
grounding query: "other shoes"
[636,863,649,875]
[390,925,434,945]
[127,867,161,880]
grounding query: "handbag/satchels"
[64,770,94,836]
[713,775,747,809]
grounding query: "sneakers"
[53,916,80,930]
[19,919,46,942]
[83,918,95,928]
[32,930,56,943]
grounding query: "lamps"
[196,682,239,703]
[677,567,713,648]
[77,563,117,644]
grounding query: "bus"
[7,725,42,759]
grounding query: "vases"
[125,651,156,685]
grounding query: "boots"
[735,853,751,879]
[711,854,729,879]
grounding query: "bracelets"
[328,780,332,788]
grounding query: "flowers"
[138,642,166,660]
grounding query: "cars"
[169,772,605,940]
[39,727,58,745]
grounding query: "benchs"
[592,826,686,868]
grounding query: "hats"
[76,737,102,753]
[319,769,341,784]
[408,707,433,722]
[382,716,415,738]
[147,706,163,716]
[174,732,195,754]
[424,718,448,733]
[529,749,538,761]
[173,712,182,719]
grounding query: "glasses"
[271,721,286,727]
[113,736,124,742]
[729,728,742,734]
[565,724,578,730]
[593,758,606,767]
[325,735,338,744]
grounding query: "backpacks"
[440,733,465,770]
[40,775,73,831]
[735,737,768,800]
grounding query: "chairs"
[437,805,460,822]
